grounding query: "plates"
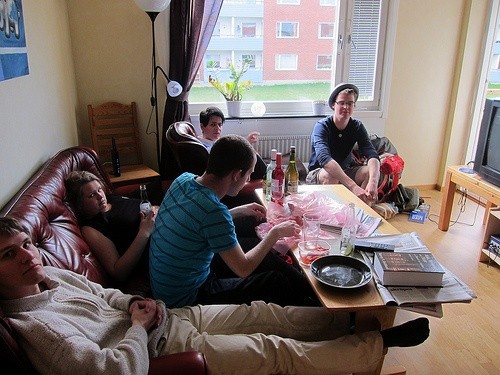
[310,255,373,289]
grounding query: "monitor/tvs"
[473,99,500,186]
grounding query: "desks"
[438,165,500,262]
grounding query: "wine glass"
[271,183,284,202]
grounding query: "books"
[372,252,446,288]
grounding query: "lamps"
[136,0,183,177]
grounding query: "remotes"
[353,240,395,253]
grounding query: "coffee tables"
[254,184,406,375]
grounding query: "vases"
[313,104,325,114]
[227,101,240,117]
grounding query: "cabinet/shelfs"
[482,210,500,266]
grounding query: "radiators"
[251,135,312,162]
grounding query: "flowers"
[207,58,253,101]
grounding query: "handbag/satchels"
[372,202,398,219]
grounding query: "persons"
[0,217,430,375]
[306,84,381,208]
[148,132,321,310]
[197,106,260,154]
[64,171,261,280]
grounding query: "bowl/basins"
[298,240,332,266]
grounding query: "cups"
[303,212,321,241]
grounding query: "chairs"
[166,122,303,197]
[88,101,163,203]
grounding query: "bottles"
[139,184,153,217]
[262,176,267,194]
[271,153,285,202]
[114,156,121,177]
[265,149,278,202]
[284,161,299,195]
[287,145,299,176]
[340,203,356,256]
[112,136,119,174]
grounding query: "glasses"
[334,100,356,107]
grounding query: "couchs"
[0,145,205,375]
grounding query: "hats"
[328,83,360,112]
